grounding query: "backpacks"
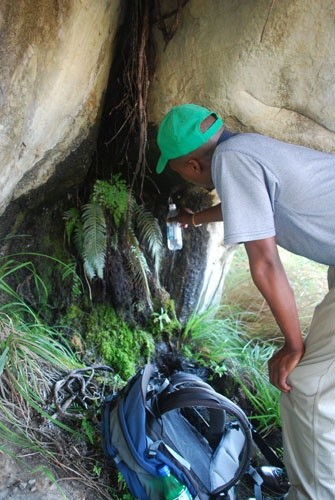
[100,361,291,500]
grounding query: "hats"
[154,102,224,174]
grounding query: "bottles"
[166,203,183,250]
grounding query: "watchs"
[191,210,203,228]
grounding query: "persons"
[156,101,335,499]
